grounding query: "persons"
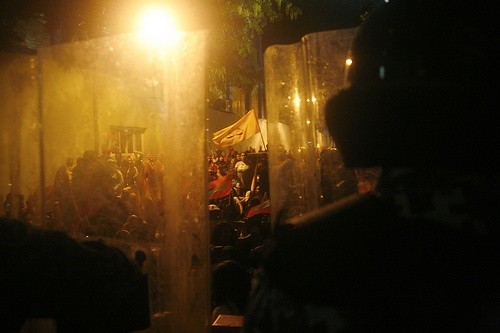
[45,124,374,281]
